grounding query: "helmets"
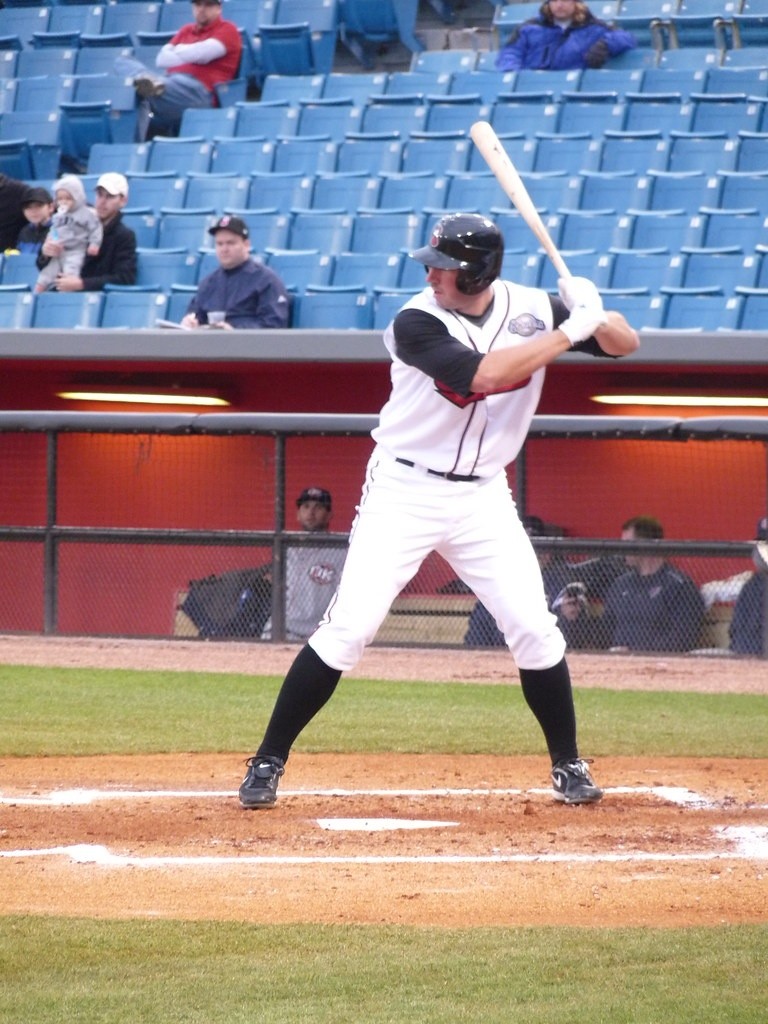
[408,214,505,295]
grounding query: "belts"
[396,456,479,482]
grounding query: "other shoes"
[132,77,167,97]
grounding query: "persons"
[35,171,138,292]
[239,213,641,809]
[0,172,31,253]
[32,175,103,293]
[260,486,348,640]
[181,214,288,330]
[18,187,56,244]
[495,0,638,71]
[114,0,242,141]
[561,515,704,652]
[727,514,768,657]
[463,515,575,648]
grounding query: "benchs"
[173,588,740,655]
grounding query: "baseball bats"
[469,119,574,278]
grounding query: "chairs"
[0,0,768,332]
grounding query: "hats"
[208,215,249,238]
[94,172,129,198]
[296,488,331,512]
[191,0,224,4]
[18,187,53,203]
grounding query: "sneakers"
[550,757,603,803]
[239,755,285,808]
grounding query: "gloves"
[586,39,606,69]
